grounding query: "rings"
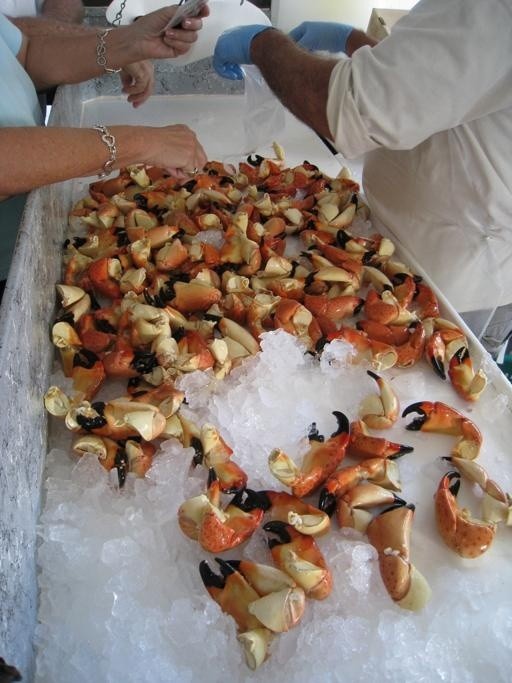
[186,166,198,176]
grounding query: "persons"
[1,0,154,108]
[212,0,512,378]
[1,0,210,283]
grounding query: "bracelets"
[95,123,116,177]
[95,25,122,75]
